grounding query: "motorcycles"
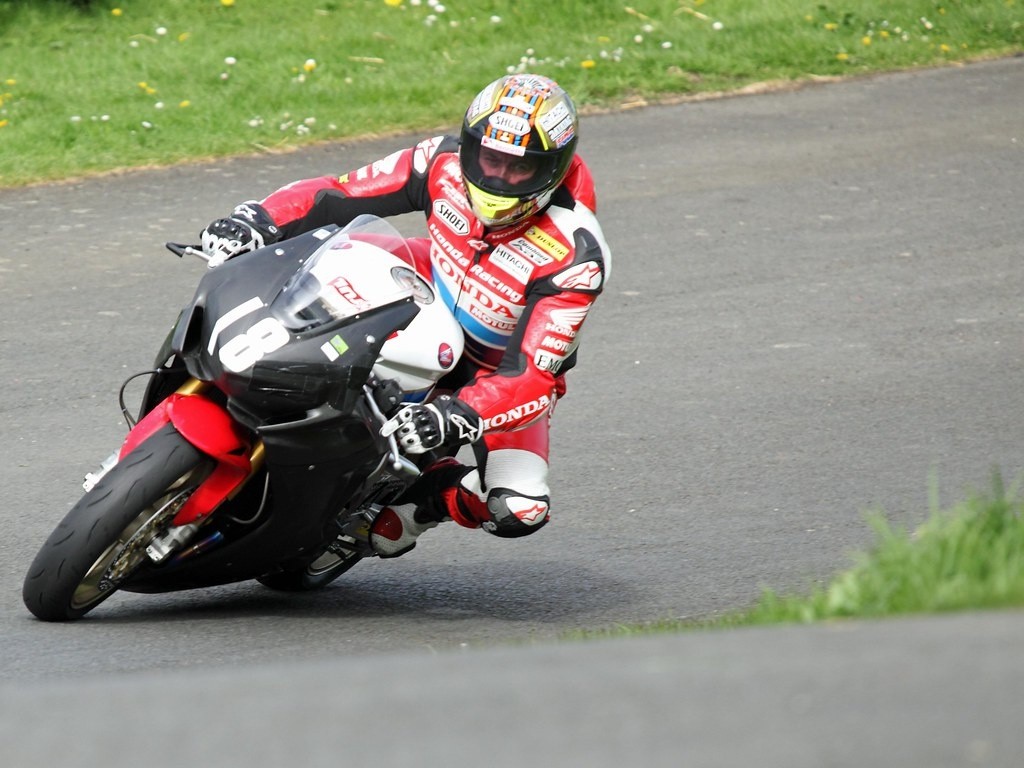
[22,214,465,623]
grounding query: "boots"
[367,456,481,557]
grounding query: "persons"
[198,74,613,560]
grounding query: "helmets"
[457,73,579,226]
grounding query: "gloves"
[379,392,483,454]
[201,200,283,269]
